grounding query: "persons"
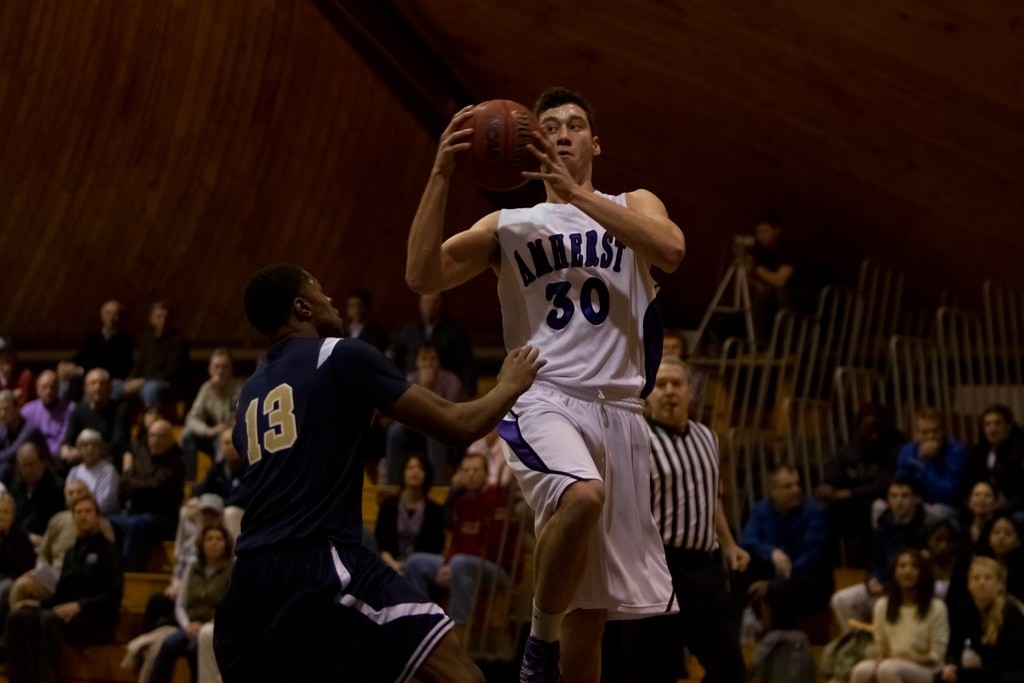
[747,212,799,315]
[733,401,1024,683]
[641,352,752,682]
[336,283,518,641]
[213,261,549,683]
[401,83,683,683]
[0,298,240,683]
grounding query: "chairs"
[0,282,1024,683]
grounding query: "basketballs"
[453,98,546,192]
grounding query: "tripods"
[689,249,757,359]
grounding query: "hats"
[194,493,224,514]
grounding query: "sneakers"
[519,635,563,683]
